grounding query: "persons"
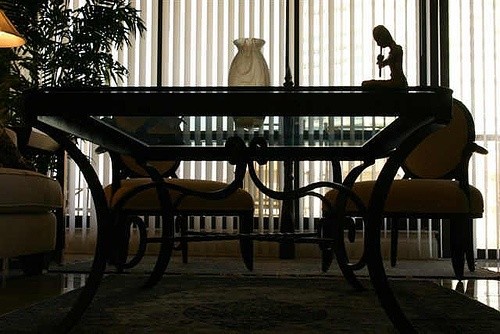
[362,25,409,93]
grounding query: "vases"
[228,38,271,129]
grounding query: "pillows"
[0,127,32,168]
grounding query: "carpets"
[1,274,500,333]
[48,253,500,280]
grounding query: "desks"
[27,127,66,264]
[17,86,454,334]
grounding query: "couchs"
[0,167,64,261]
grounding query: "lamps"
[0,9,27,48]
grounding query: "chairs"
[94,116,254,275]
[321,98,488,283]
